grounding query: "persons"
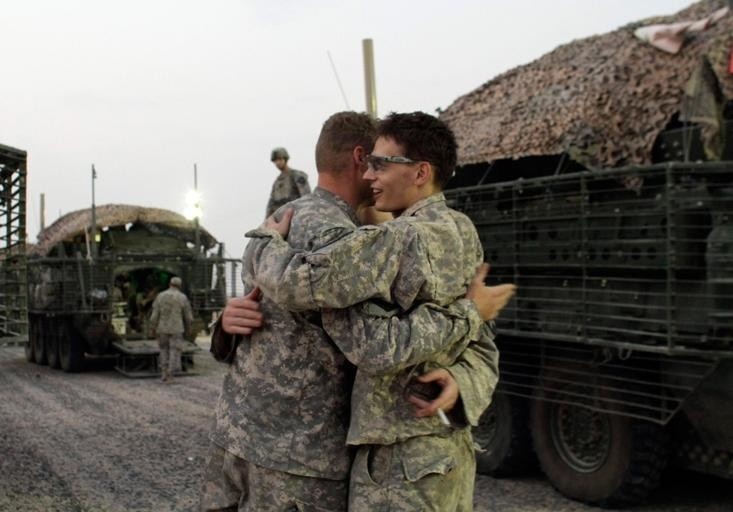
[148,276,193,383]
[245,112,482,512]
[265,147,311,218]
[204,111,518,512]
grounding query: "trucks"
[25,204,226,374]
[438,0,732,505]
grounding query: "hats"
[170,277,182,286]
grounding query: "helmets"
[270,147,289,161]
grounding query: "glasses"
[365,155,415,172]
